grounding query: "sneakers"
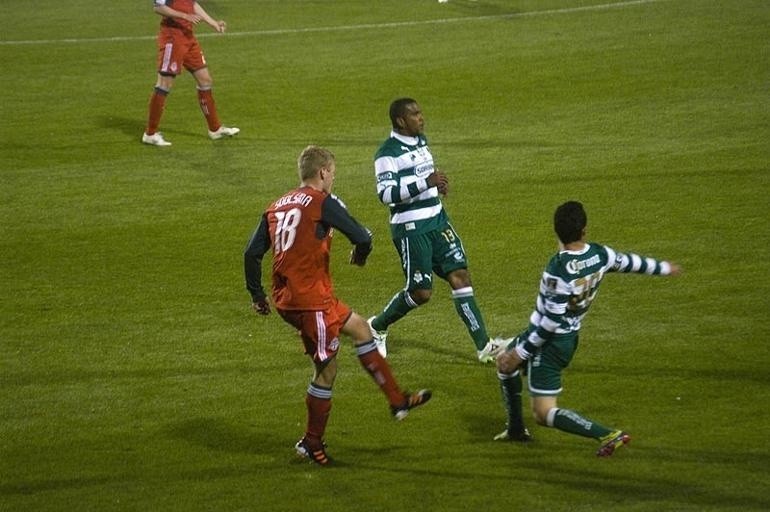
[208,126,240,140]
[294,438,332,466]
[391,389,431,421]
[476,337,515,362]
[142,133,172,146]
[596,431,629,457]
[366,316,389,359]
[494,428,530,441]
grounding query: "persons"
[367,98,519,365]
[243,144,434,470]
[141,0,242,147]
[493,201,681,459]
